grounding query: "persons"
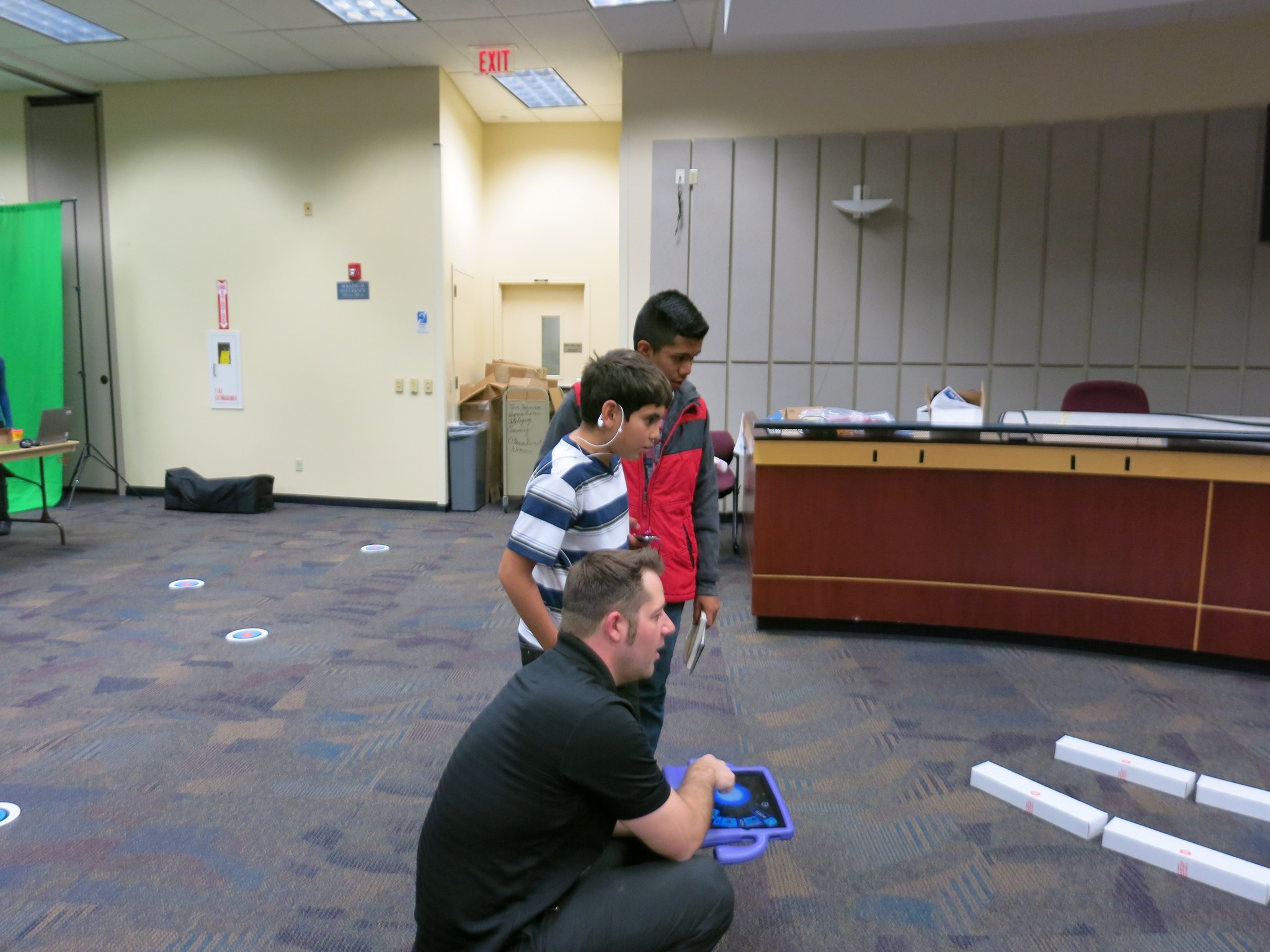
[533,286,722,756]
[496,350,677,719]
[0,353,13,537]
[414,547,737,952]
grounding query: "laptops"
[31,407,73,446]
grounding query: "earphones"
[597,412,604,427]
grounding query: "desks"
[750,420,1270,671]
[0,439,80,546]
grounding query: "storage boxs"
[782,406,823,421]
[1101,815,1270,906]
[1053,734,1197,799]
[1195,773,1270,822]
[923,379,986,439]
[969,760,1109,840]
[0,426,23,445]
[459,359,567,506]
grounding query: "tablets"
[660,765,795,847]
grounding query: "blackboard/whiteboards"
[502,399,550,496]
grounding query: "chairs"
[708,429,742,554]
[1060,380,1150,414]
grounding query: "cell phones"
[632,534,658,542]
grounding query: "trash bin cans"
[448,420,489,512]
